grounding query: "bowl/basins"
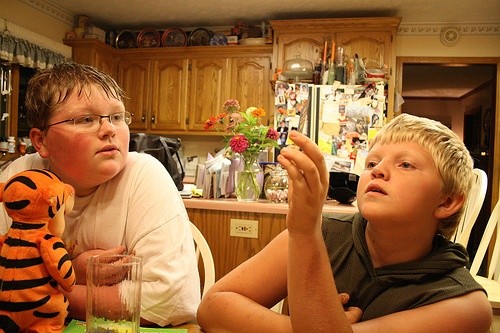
[239,37,266,46]
[265,168,290,204]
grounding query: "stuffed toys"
[0,168,76,333]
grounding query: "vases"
[234,151,265,203]
[263,170,290,204]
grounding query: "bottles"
[0,136,19,155]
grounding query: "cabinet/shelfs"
[64,14,404,150]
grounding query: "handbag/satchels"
[129,132,185,191]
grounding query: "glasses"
[39,111,134,132]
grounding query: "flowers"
[203,99,282,199]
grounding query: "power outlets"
[230,218,259,239]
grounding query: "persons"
[277,83,307,145]
[196,114,493,333]
[0,63,201,327]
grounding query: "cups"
[19,142,27,153]
[313,70,319,85]
[85,255,142,333]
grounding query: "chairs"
[448,166,500,317]
[189,220,216,302]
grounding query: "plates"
[115,26,228,49]
